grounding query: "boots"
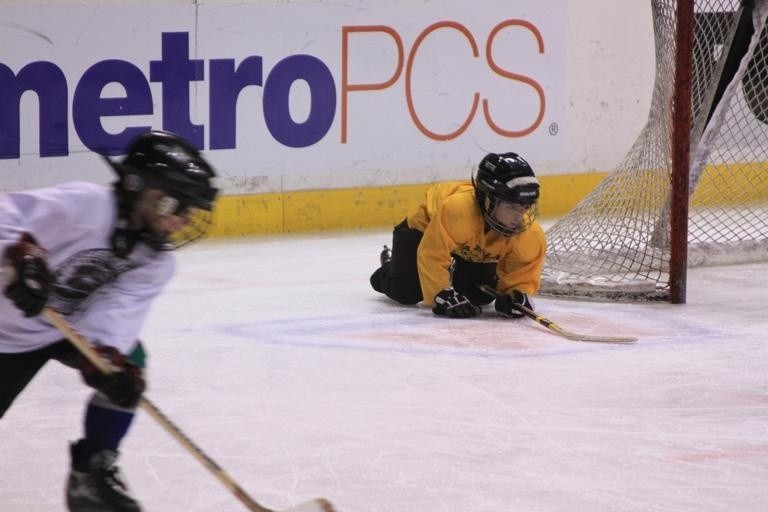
[68,441,138,511]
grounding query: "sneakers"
[380,248,392,264]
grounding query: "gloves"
[1,232,53,318]
[80,342,143,406]
[495,291,536,319]
[432,287,481,318]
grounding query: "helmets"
[99,130,217,258]
[471,151,540,237]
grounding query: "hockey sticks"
[480,285,637,342]
[1,266,333,512]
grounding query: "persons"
[369,151,547,319]
[0,128,222,512]
